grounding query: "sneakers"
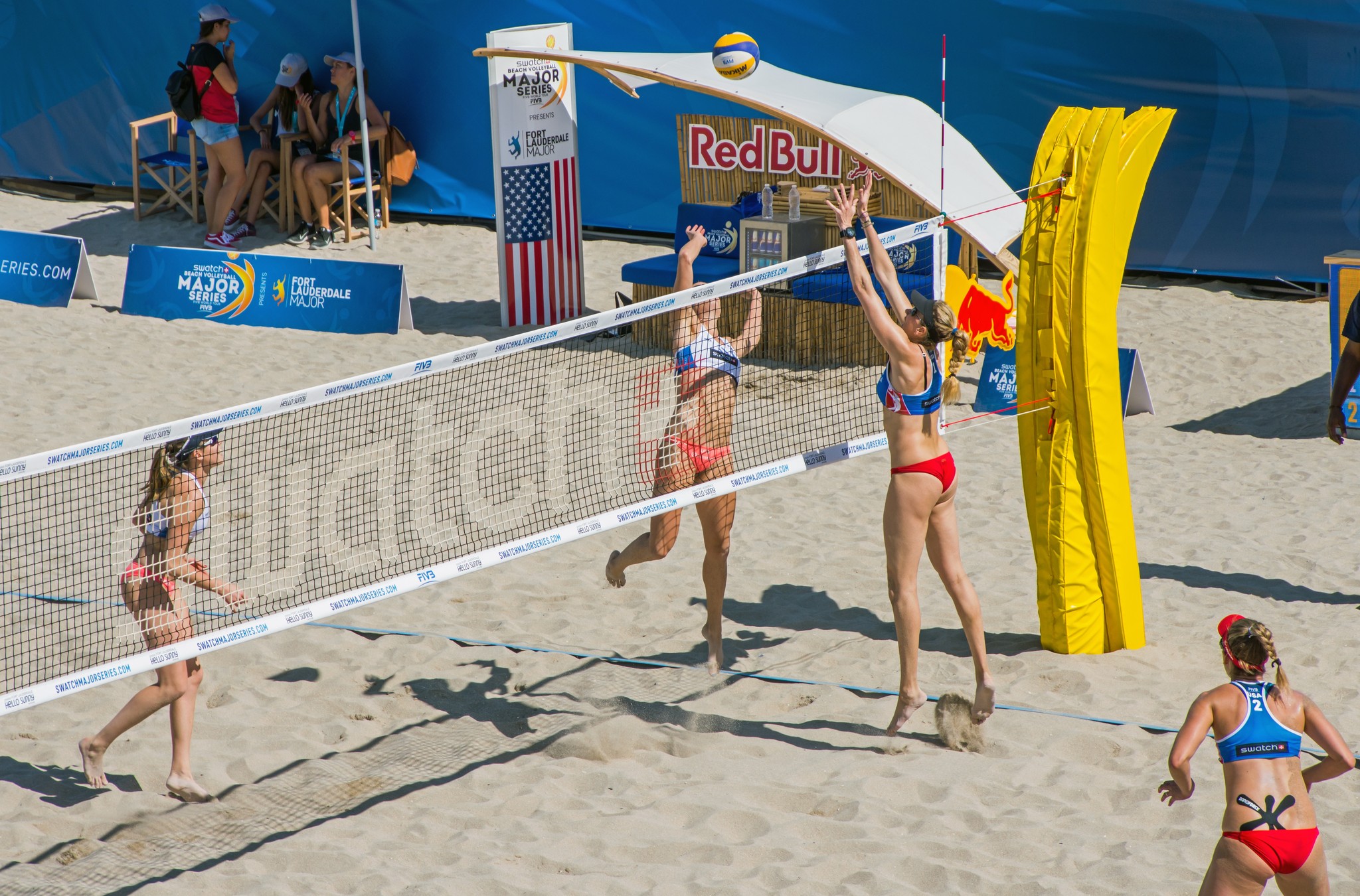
[285,220,317,244]
[224,208,242,229]
[222,230,242,243]
[204,230,238,251]
[230,223,257,239]
[309,226,336,250]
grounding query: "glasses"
[1219,638,1224,650]
[910,306,925,326]
[183,435,217,456]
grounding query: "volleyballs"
[712,31,761,82]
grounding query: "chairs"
[129,108,390,243]
[621,201,962,367]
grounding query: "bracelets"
[259,130,266,136]
[1329,405,1341,409]
[858,211,874,229]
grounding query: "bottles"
[374,209,382,239]
[233,96,239,126]
[788,185,800,220]
[762,183,773,219]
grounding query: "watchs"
[839,227,856,238]
[349,130,356,145]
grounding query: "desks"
[1324,249,1360,429]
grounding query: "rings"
[837,201,843,205]
[1166,791,1170,797]
[852,204,856,207]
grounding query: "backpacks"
[165,46,215,122]
[382,114,420,187]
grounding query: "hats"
[169,427,224,463]
[198,3,242,23]
[324,51,366,72]
[911,289,941,344]
[275,52,308,87]
[1218,614,1269,671]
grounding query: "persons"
[1158,614,1356,896]
[605,225,762,673]
[286,51,388,249]
[1327,290,1360,445]
[826,169,993,736]
[79,428,247,802]
[223,52,322,239]
[186,3,246,251]
